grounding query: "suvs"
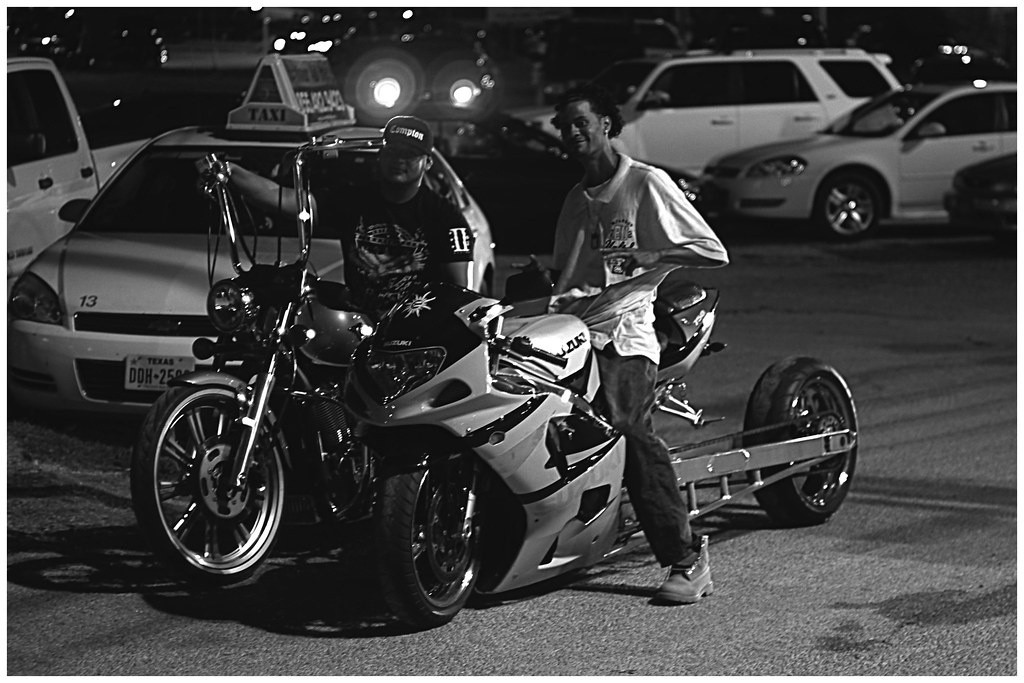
[497,43,904,204]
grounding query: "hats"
[381,115,433,157]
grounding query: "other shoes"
[654,534,714,604]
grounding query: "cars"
[944,146,1018,239]
[843,22,1008,88]
[7,29,351,288]
[700,80,1018,242]
[7,118,498,430]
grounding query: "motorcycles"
[342,249,860,625]
[129,133,557,591]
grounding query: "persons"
[200,116,475,320]
[510,80,730,605]
